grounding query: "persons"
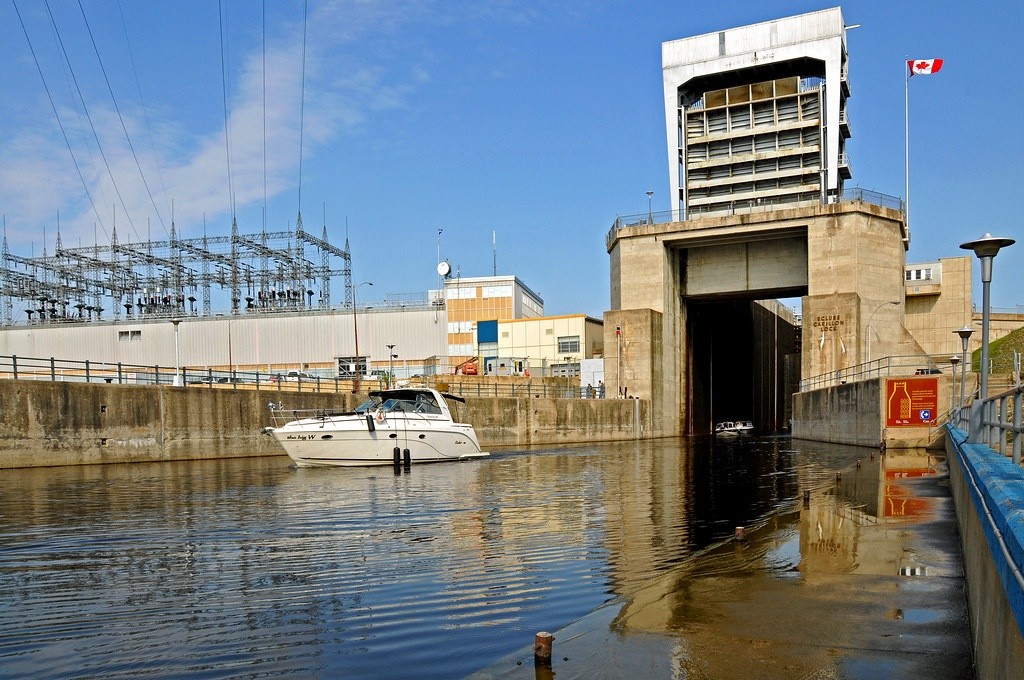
[586,380,603,399]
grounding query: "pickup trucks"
[361,369,396,380]
[189,376,218,385]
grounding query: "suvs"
[217,377,246,384]
[286,371,317,384]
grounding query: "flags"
[907,58,943,78]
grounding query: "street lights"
[646,189,654,224]
[169,317,183,384]
[960,232,1015,400]
[387,342,398,389]
[818,168,827,205]
[867,299,902,377]
[352,280,373,378]
[227,309,252,384]
[949,355,961,412]
[952,324,976,405]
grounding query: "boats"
[735,420,753,436]
[716,422,737,438]
[261,381,488,469]
